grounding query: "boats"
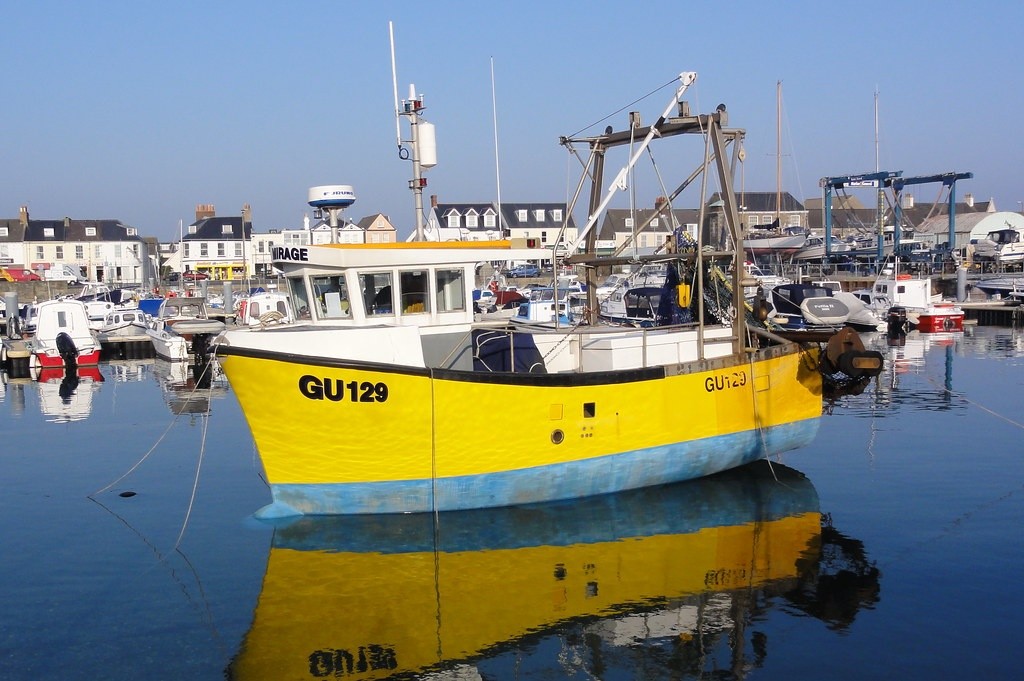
[207,71,884,520]
[145,297,226,363]
[592,273,668,321]
[856,225,923,259]
[511,287,583,323]
[741,283,880,331]
[243,289,295,326]
[872,256,964,336]
[970,228,1024,272]
[793,236,854,260]
[30,299,103,368]
[103,308,150,335]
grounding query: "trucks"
[54,263,89,282]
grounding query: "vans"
[4,269,40,282]
[45,270,77,285]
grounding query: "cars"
[182,270,208,280]
[507,263,541,277]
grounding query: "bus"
[194,262,250,280]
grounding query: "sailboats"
[743,79,810,253]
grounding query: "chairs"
[374,286,392,313]
